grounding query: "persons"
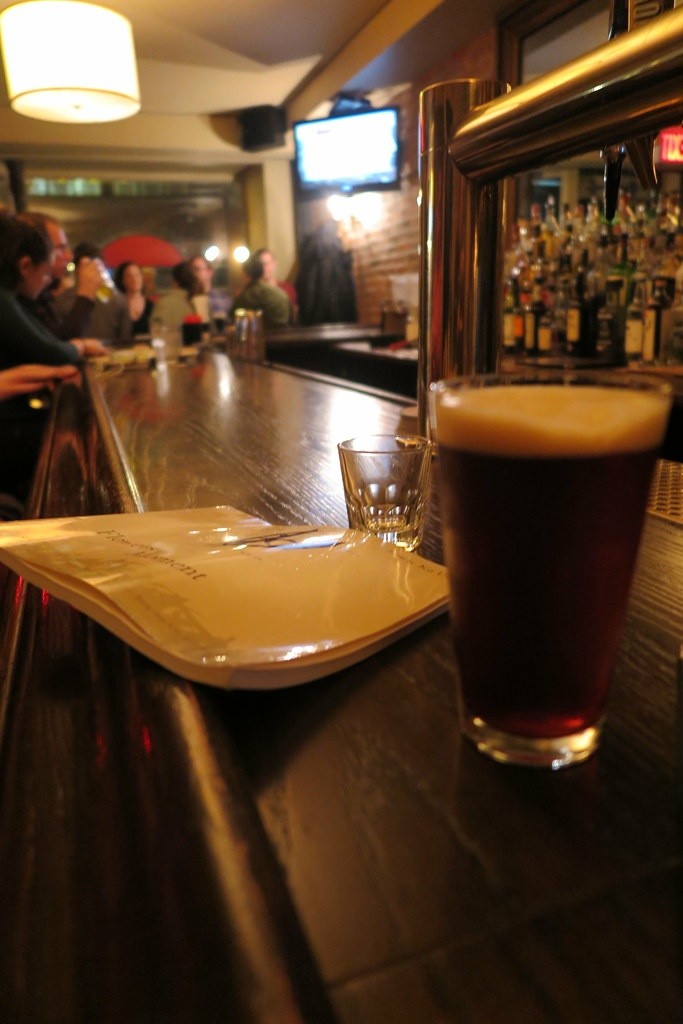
[1,206,299,535]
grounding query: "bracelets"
[80,337,88,356]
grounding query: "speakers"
[241,106,285,152]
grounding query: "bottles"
[382,300,408,333]
[501,189,683,371]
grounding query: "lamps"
[0,0,143,124]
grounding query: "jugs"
[232,308,265,362]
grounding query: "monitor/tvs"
[293,104,400,196]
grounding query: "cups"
[153,328,178,374]
[424,372,671,768]
[339,435,433,553]
